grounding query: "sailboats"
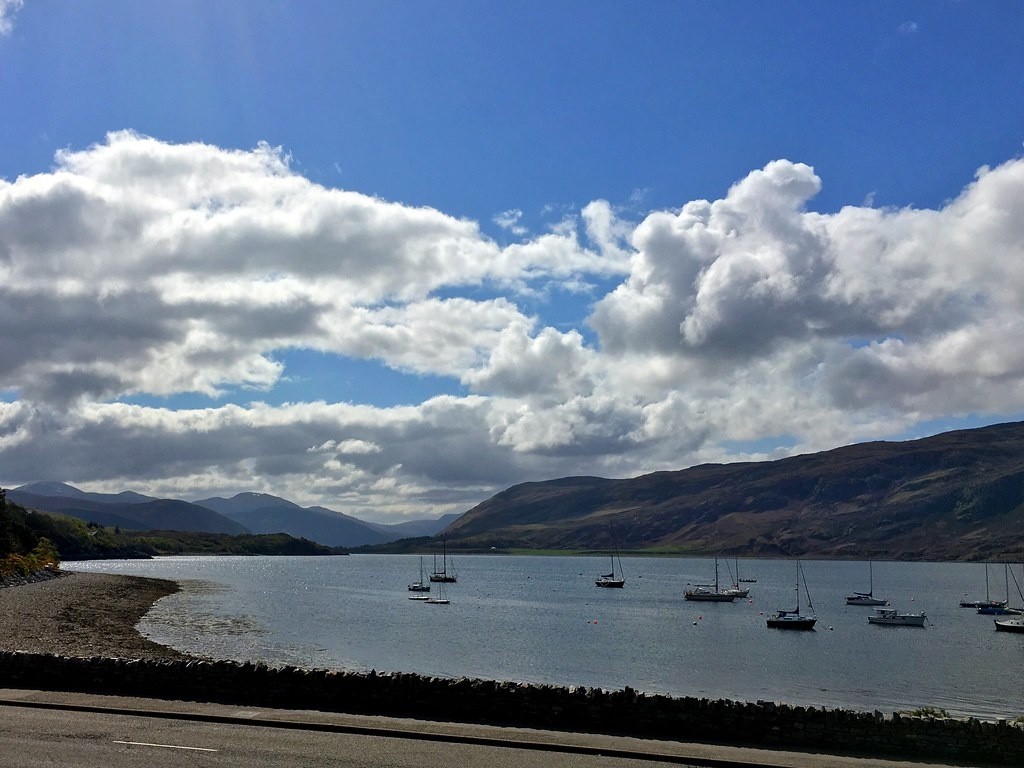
[595,528,626,588]
[428,538,458,583]
[407,556,431,592]
[768,558,818,631]
[846,557,888,606]
[718,557,750,599]
[424,583,450,604]
[956,557,1024,616]
[684,555,736,602]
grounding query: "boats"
[408,596,430,600]
[867,607,928,626]
[992,618,1024,631]
